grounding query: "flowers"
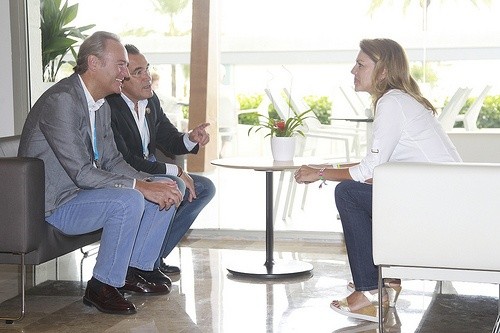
[237,86,332,137]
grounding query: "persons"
[103,43,216,285]
[17,31,187,316]
[295,38,463,324]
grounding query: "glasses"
[128,66,153,78]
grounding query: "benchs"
[372,132,500,333]
[0,135,185,325]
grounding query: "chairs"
[155,89,189,132]
[262,84,372,229]
[438,83,493,133]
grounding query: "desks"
[178,101,189,107]
[210,158,327,279]
[323,116,374,161]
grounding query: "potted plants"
[40,0,97,92]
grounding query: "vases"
[271,136,295,162]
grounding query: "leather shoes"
[117,274,170,296]
[159,258,181,275]
[134,267,172,285]
[83,280,137,314]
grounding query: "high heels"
[329,287,389,323]
[348,279,402,308]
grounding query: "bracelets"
[178,166,183,177]
[332,164,340,168]
[319,168,325,180]
